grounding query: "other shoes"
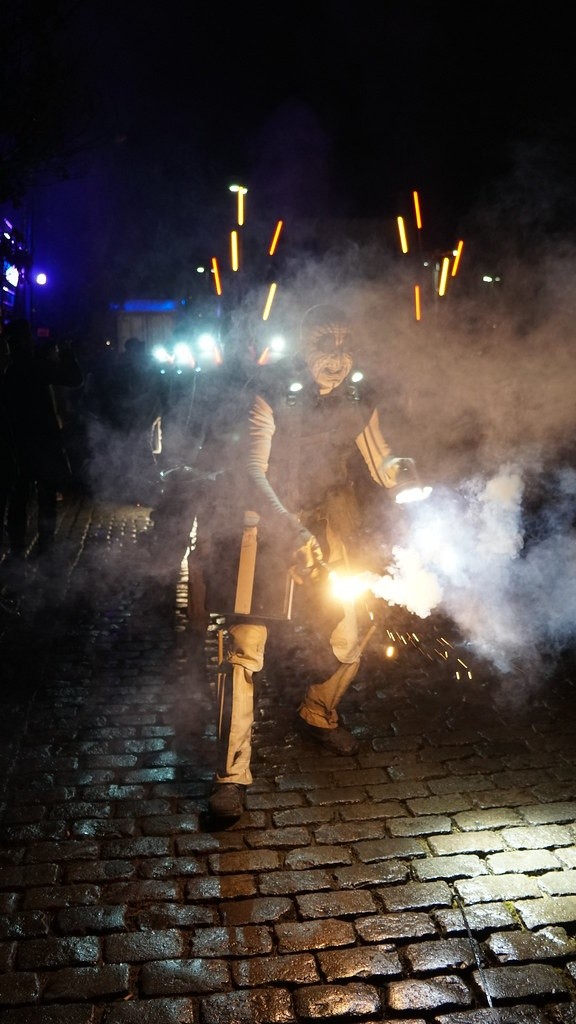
[295,716,360,756]
[209,784,246,816]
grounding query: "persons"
[0,302,413,820]
